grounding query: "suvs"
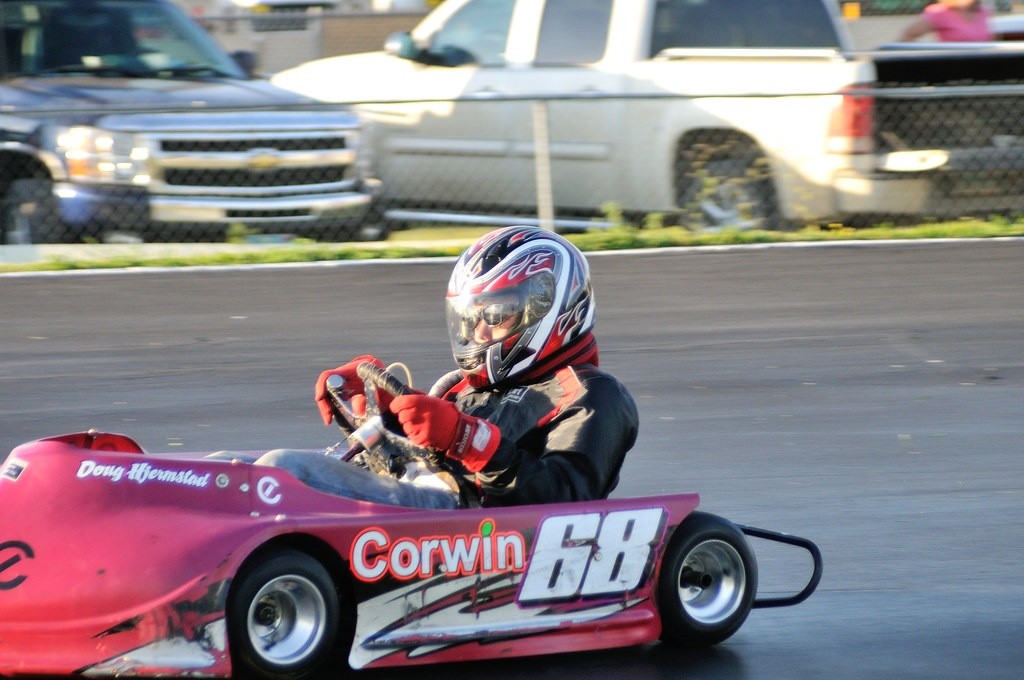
[0,1,389,244]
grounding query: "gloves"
[388,385,501,474]
[315,354,395,425]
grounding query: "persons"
[201,225,639,510]
[897,0,995,42]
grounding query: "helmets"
[445,226,596,387]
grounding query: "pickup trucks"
[263,0,1023,235]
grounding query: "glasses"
[460,303,526,332]
